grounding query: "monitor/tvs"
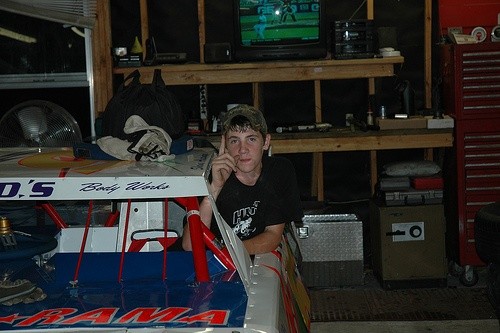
[234,0,327,62]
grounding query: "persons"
[181,103,304,255]
[271,0,296,25]
[255,11,267,40]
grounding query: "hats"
[222,105,267,137]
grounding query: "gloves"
[129,129,172,161]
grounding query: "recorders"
[331,0,377,59]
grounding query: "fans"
[0,101,83,146]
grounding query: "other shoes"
[1,288,46,305]
[0,279,35,300]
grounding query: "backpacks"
[102,68,188,139]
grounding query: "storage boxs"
[368,202,447,289]
[292,213,365,288]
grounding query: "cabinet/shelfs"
[100,0,453,200]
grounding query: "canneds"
[379,105,386,117]
